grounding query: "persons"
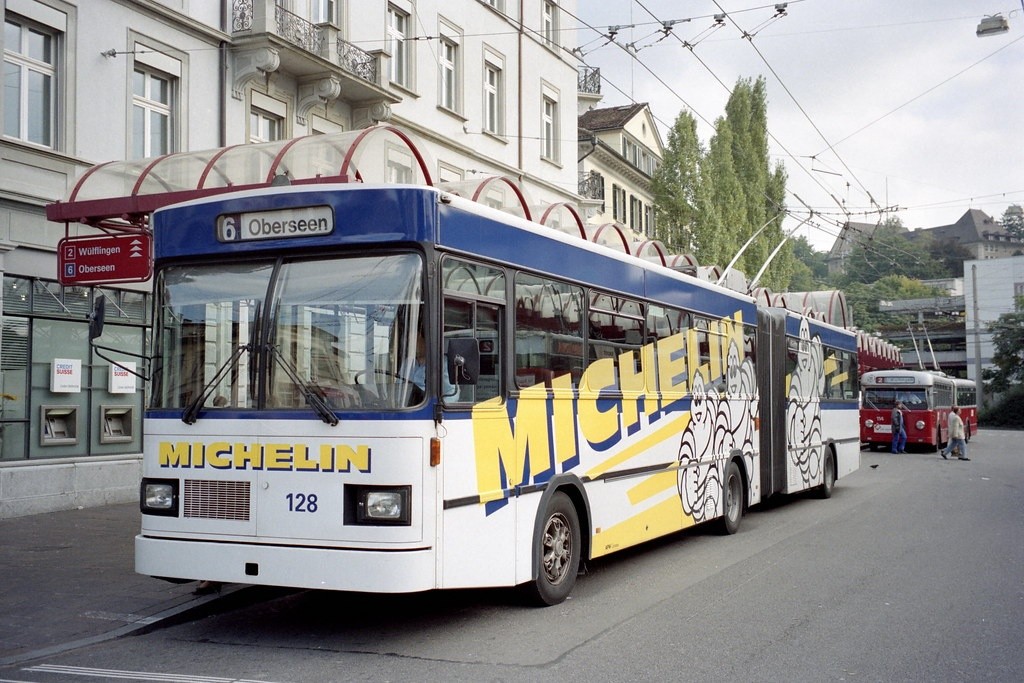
[900,390,922,404]
[395,324,460,403]
[890,400,907,454]
[941,405,971,461]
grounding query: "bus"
[860,369,978,452]
[128,184,860,607]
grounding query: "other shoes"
[940,450,944,453]
[893,452,898,454]
[952,453,961,456]
[941,453,948,459]
[899,449,906,454]
[958,457,969,460]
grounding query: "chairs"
[555,369,585,389]
[517,368,555,388]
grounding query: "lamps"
[976,15,1011,38]
